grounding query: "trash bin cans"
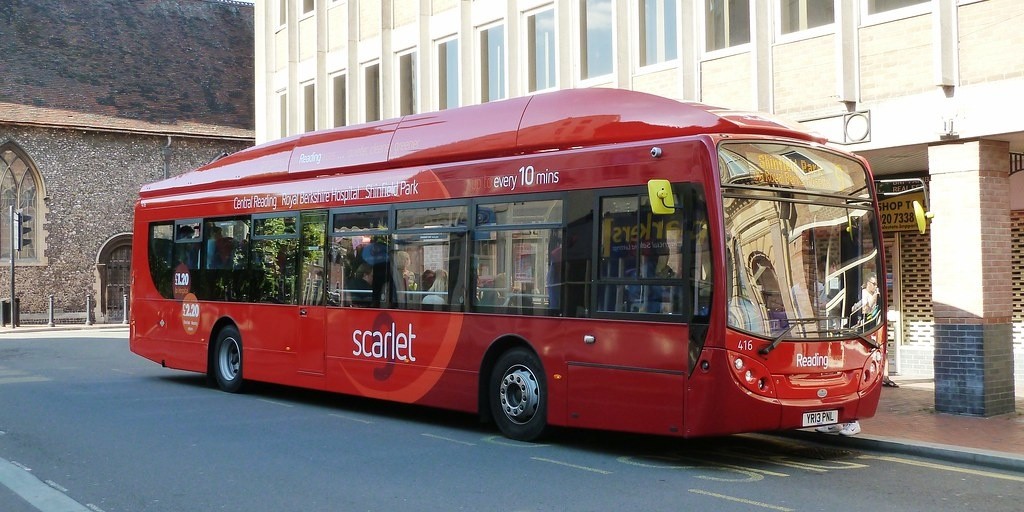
[0,297,21,327]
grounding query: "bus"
[128,87,884,447]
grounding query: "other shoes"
[883,376,899,387]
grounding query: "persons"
[547,248,562,308]
[862,272,899,388]
[480,273,534,316]
[348,239,449,309]
[792,255,840,338]
[624,239,709,317]
[179,226,242,269]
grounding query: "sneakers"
[839,420,861,436]
[815,424,843,432]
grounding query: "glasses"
[869,281,877,286]
